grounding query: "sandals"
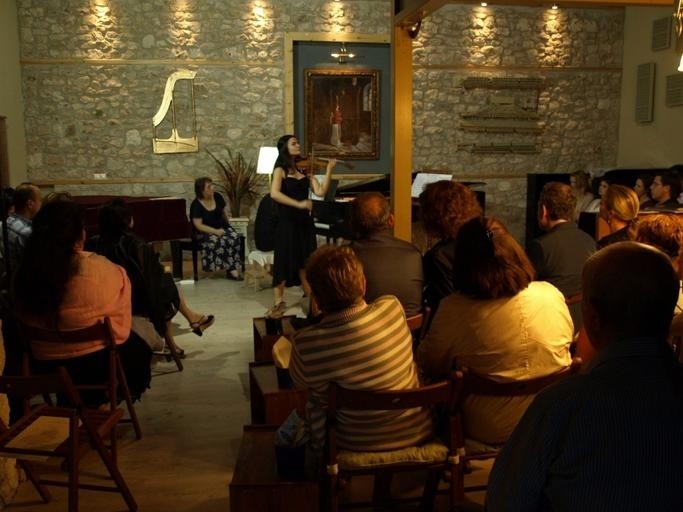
[190,315,215,336]
[164,347,186,363]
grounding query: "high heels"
[227,271,244,281]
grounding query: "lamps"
[328,41,359,63]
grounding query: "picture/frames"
[301,67,382,164]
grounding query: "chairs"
[230,291,591,511]
[0,283,183,512]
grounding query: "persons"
[307,191,424,351]
[268,135,336,309]
[189,177,242,281]
[2,182,42,250]
[414,217,576,448]
[526,165,683,371]
[484,241,683,511]
[329,105,342,146]
[13,191,166,411]
[286,244,437,488]
[419,180,484,335]
[84,199,213,361]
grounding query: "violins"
[296,157,355,174]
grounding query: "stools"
[173,233,247,281]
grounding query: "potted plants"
[197,144,270,261]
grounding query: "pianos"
[70,196,197,278]
[311,173,487,257]
